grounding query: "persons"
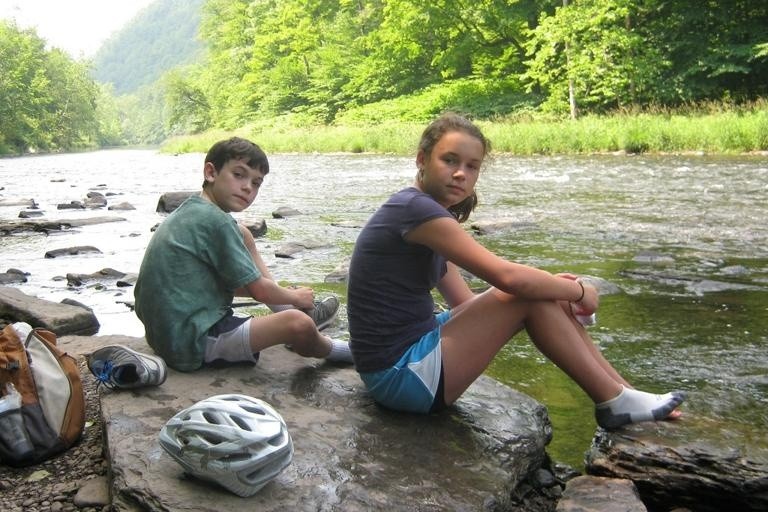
[347,112,684,431]
[135,137,354,372]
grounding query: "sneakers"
[86,343,169,391]
[284,294,340,349]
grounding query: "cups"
[0,399,35,458]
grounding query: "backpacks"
[0,322,85,469]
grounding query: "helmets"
[157,393,295,498]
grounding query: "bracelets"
[573,279,585,303]
